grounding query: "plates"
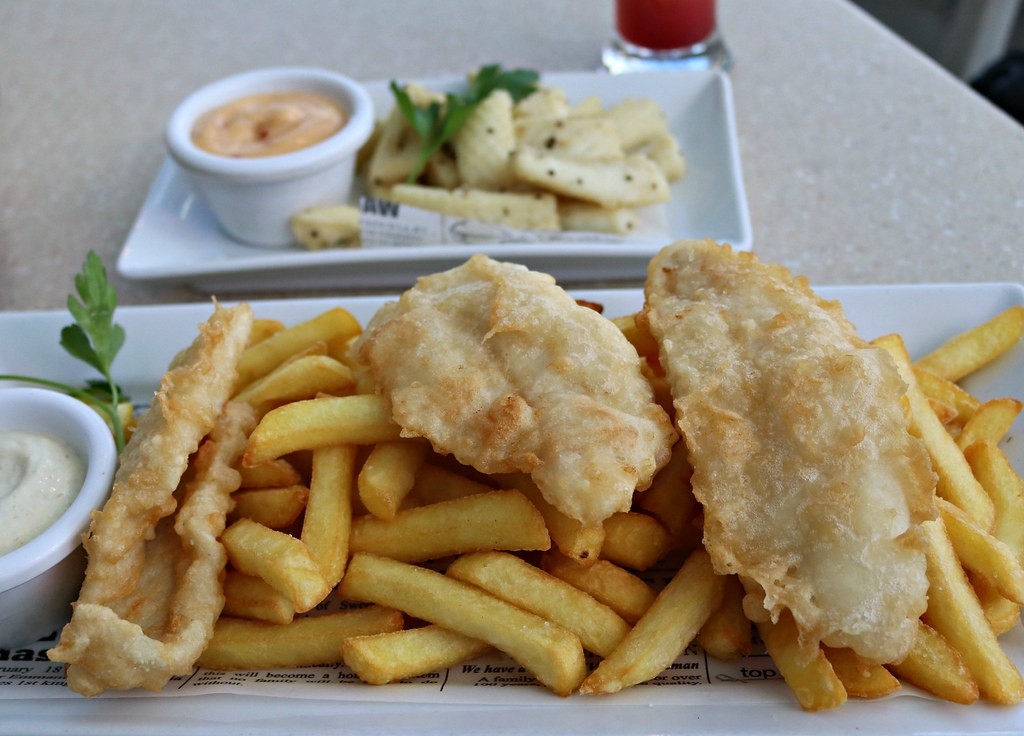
[112,64,752,295]
[0,279,1024,736]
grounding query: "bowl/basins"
[161,67,377,248]
[0,384,121,651]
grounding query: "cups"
[602,0,734,77]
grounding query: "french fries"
[87,308,1023,714]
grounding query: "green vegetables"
[0,250,125,462]
[386,61,542,187]
